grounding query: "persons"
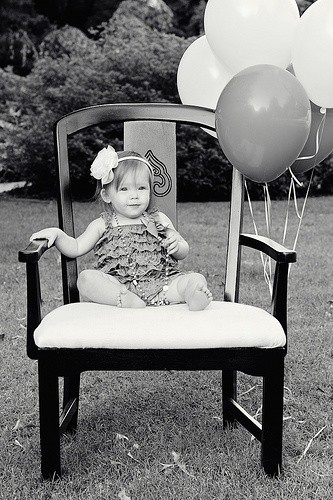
[28,151,214,312]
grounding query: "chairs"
[16,105,298,482]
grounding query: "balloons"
[203,0,301,71]
[292,0,333,115]
[215,63,311,183]
[177,34,235,138]
[279,65,333,175]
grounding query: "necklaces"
[112,208,171,306]
